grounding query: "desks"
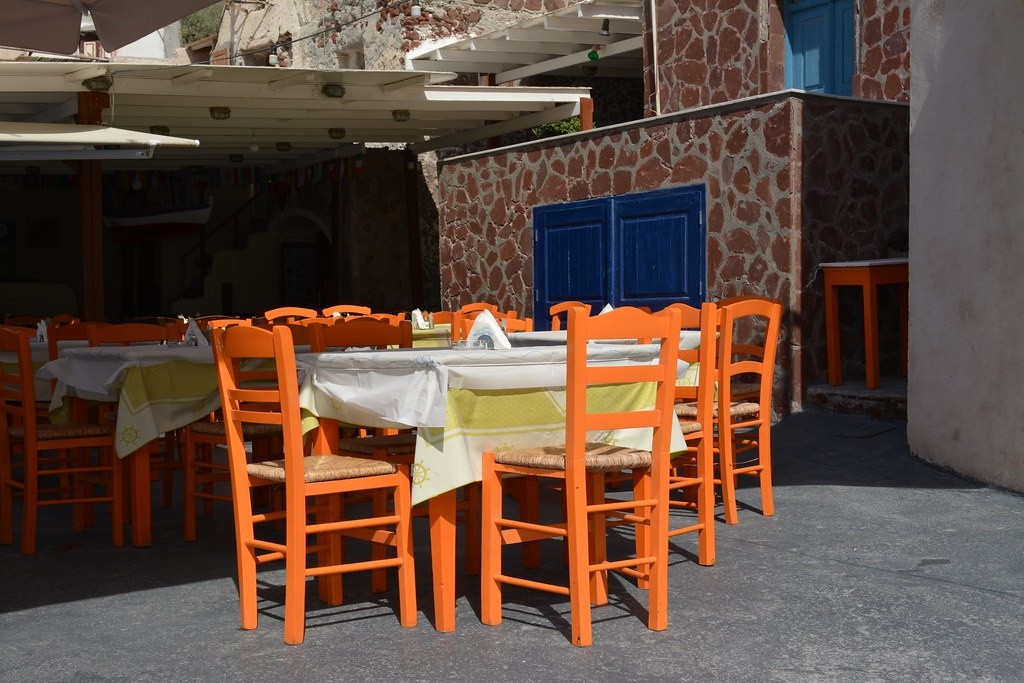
[290,343,688,632]
[500,320,720,507]
[385,321,465,349]
[32,344,307,547]
[818,257,909,389]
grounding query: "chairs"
[0,292,783,648]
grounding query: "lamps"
[599,19,611,37]
